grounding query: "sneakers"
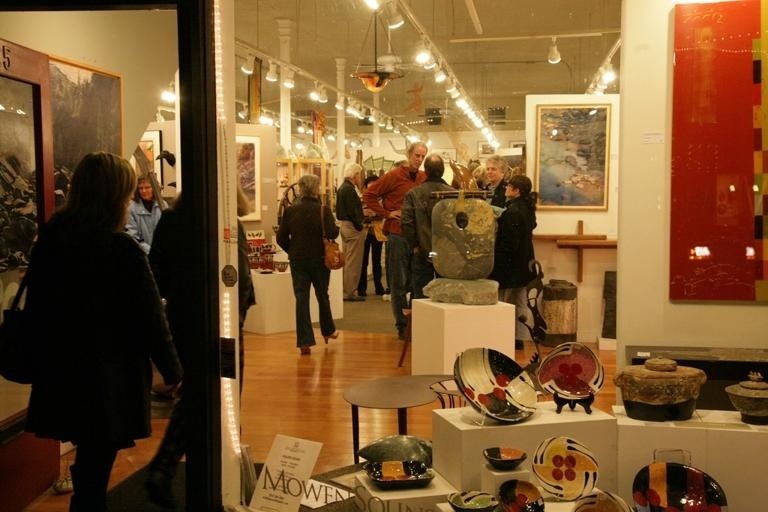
[344,289,392,302]
[53,478,73,493]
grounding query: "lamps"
[590,60,614,94]
[385,0,502,150]
[547,43,561,65]
[242,53,402,135]
[237,106,357,148]
[161,81,176,104]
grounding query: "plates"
[532,435,729,512]
[453,341,605,422]
[362,459,435,490]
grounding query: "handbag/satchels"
[324,239,343,269]
[1,307,35,384]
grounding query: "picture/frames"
[477,142,498,158]
[535,105,611,211]
[49,53,124,213]
[432,148,457,163]
[129,130,162,186]
[510,141,527,150]
[235,136,261,222]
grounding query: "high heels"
[323,332,338,344]
[301,347,311,354]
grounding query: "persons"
[123,172,169,255]
[140,166,259,512]
[19,150,186,512]
[334,162,385,302]
[363,142,457,340]
[237,143,255,194]
[275,173,345,356]
[473,156,538,351]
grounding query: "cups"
[262,244,272,251]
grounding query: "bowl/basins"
[446,479,545,512]
[483,447,528,470]
[273,261,290,272]
